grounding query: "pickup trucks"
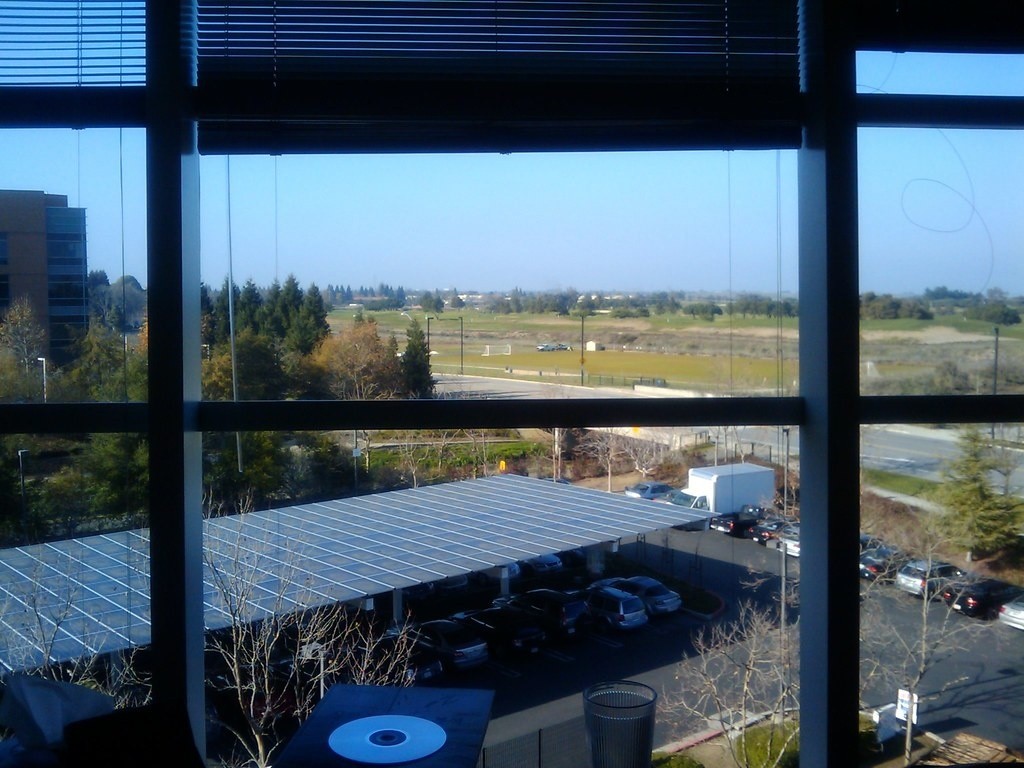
[709,504,776,538]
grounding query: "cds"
[329,715,446,764]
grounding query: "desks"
[274,682,496,768]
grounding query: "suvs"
[893,558,967,602]
[492,588,593,636]
[564,583,649,632]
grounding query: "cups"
[583,680,657,768]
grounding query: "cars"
[749,517,799,546]
[543,477,571,485]
[859,547,913,585]
[201,603,551,743]
[536,342,568,352]
[944,577,1024,617]
[999,593,1024,632]
[775,521,800,558]
[624,482,682,505]
[860,533,884,556]
[590,576,682,616]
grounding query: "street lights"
[766,539,788,723]
[458,317,463,374]
[578,313,596,386]
[781,426,791,516]
[201,344,211,362]
[18,450,29,546]
[37,358,48,403]
[991,327,1000,440]
[426,316,434,366]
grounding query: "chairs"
[63,699,206,768]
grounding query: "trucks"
[667,462,777,532]
